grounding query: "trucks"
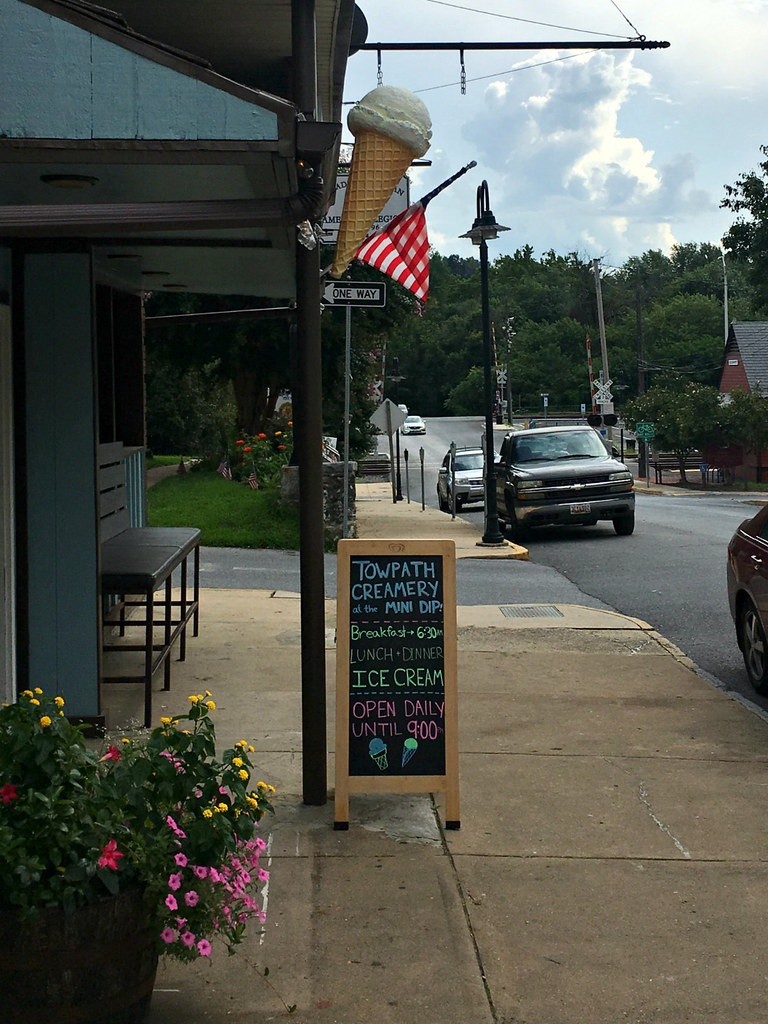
[398,404,410,415]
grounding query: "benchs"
[637,445,744,485]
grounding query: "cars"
[726,503,768,699]
[400,416,426,435]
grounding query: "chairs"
[96,437,202,730]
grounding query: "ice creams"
[332,85,433,280]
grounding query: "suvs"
[436,446,503,513]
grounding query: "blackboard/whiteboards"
[335,539,459,794]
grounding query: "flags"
[216,462,232,480]
[248,473,259,490]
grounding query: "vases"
[0,868,160,1024]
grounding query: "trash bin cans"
[626,439,636,453]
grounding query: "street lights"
[459,179,511,548]
[718,255,729,346]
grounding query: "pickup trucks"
[483,425,636,536]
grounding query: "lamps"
[294,117,342,154]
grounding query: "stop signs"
[496,391,500,400]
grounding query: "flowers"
[0,684,281,957]
